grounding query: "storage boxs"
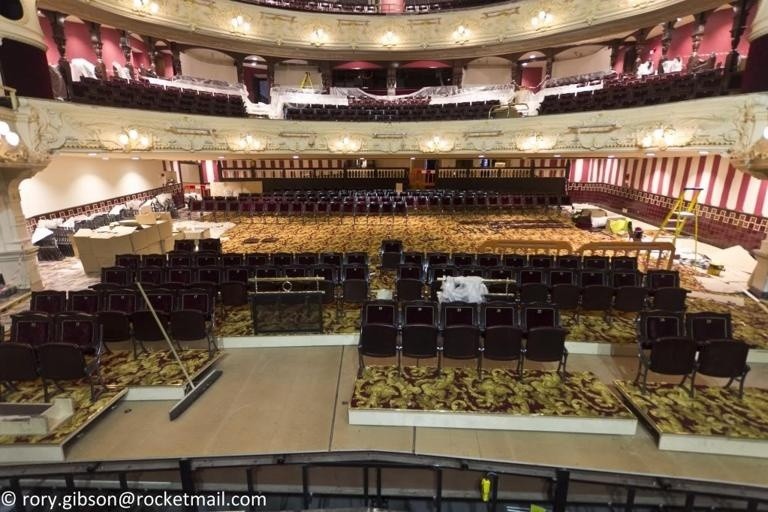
[74,204,210,275]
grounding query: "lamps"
[229,14,251,37]
[118,128,150,153]
[449,25,474,45]
[128,0,159,19]
[530,10,556,34]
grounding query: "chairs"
[187,188,575,220]
[56,59,746,123]
[0,234,755,405]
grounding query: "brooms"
[133,279,224,422]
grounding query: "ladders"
[643,187,704,260]
